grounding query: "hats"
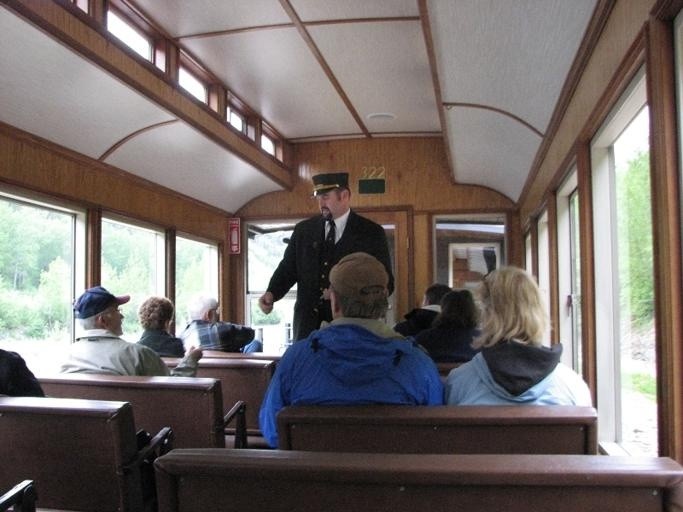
[74,285,130,319]
[330,252,388,301]
[310,172,349,194]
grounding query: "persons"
[262,173,395,348]
[410,288,480,365]
[445,265,596,408]
[0,349,47,399]
[256,252,444,448]
[135,297,186,358]
[394,284,451,335]
[59,286,203,377]
[189,297,263,353]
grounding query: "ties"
[325,221,335,242]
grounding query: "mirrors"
[431,212,510,292]
